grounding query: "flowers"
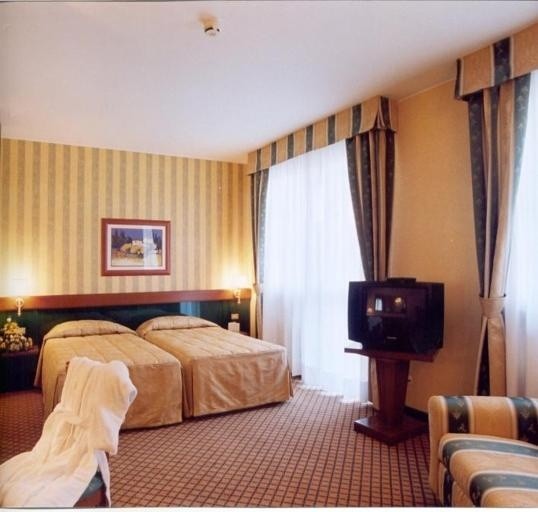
[0,316,35,352]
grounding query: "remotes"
[387,277,417,281]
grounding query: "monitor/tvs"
[347,281,445,354]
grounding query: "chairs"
[0,361,122,508]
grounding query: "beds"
[32,314,296,432]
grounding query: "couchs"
[426,394,537,509]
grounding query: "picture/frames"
[100,215,172,276]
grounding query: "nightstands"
[0,343,39,393]
